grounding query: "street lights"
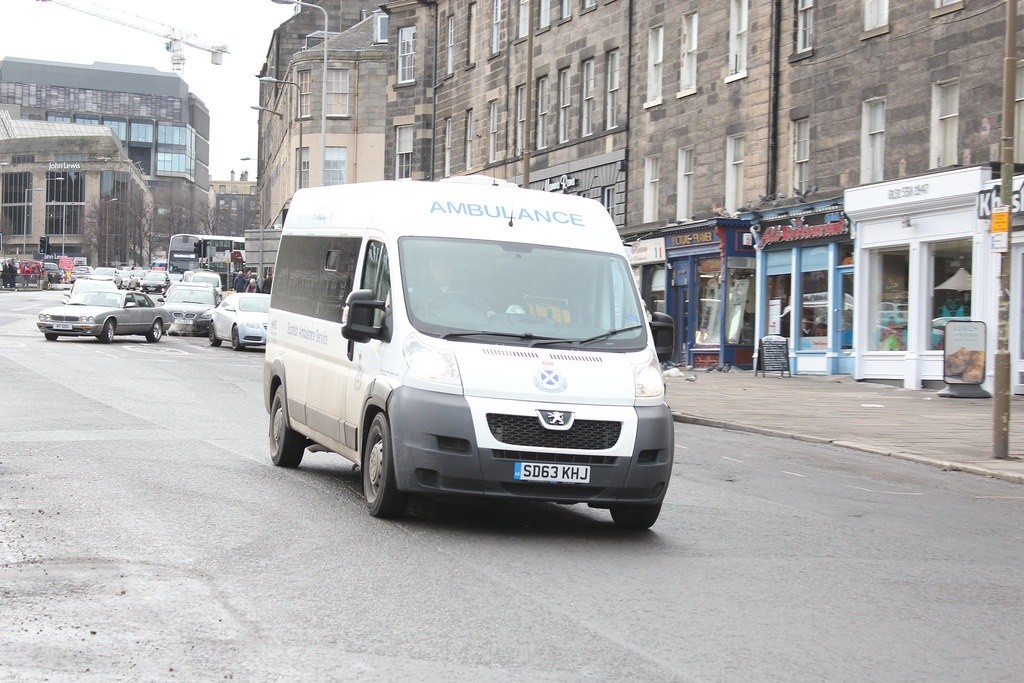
[23,188,43,258]
[106,198,118,267]
[52,200,65,257]
[44,177,64,236]
[259,76,303,188]
[273,0,327,184]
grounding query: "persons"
[417,266,495,321]
[1,259,17,288]
[21,262,32,287]
[234,271,272,294]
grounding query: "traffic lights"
[38,236,46,254]
[48,236,52,255]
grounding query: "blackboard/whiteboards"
[760,338,790,371]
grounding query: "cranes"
[36,0,232,75]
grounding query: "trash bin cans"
[40,280,48,291]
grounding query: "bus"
[166,233,234,284]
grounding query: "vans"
[260,174,677,528]
[190,273,223,294]
[150,261,168,273]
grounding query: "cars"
[140,272,170,293]
[39,263,61,283]
[36,290,171,342]
[88,267,123,289]
[63,279,118,304]
[71,266,95,283]
[162,282,220,306]
[135,270,147,285]
[118,270,138,292]
[208,292,269,350]
[158,288,222,336]
